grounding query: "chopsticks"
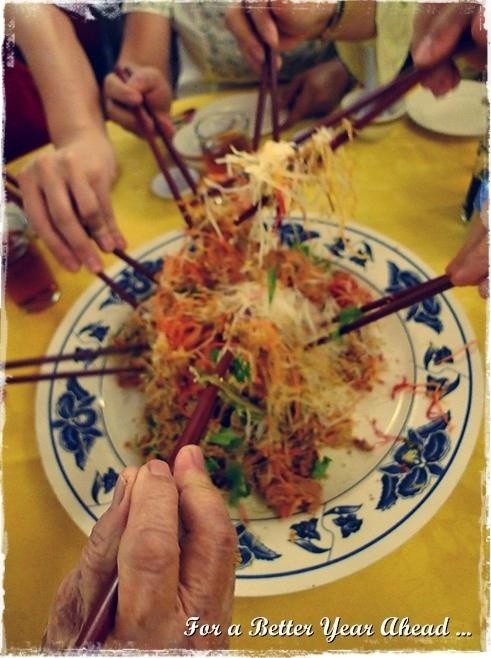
[190,34,476,226]
[71,347,232,655]
[3,171,159,308]
[253,48,285,231]
[3,344,147,382]
[304,267,489,350]
[113,65,208,228]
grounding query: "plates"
[405,78,489,136]
[34,207,480,598]
[151,166,199,198]
[173,91,288,158]
[337,88,406,124]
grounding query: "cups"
[3,210,62,317]
[194,109,253,182]
[459,135,489,221]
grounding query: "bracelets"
[315,1,346,42]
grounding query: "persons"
[409,0,486,103]
[43,441,240,651]
[103,2,357,142]
[2,3,129,273]
[442,203,490,300]
[221,2,480,96]
